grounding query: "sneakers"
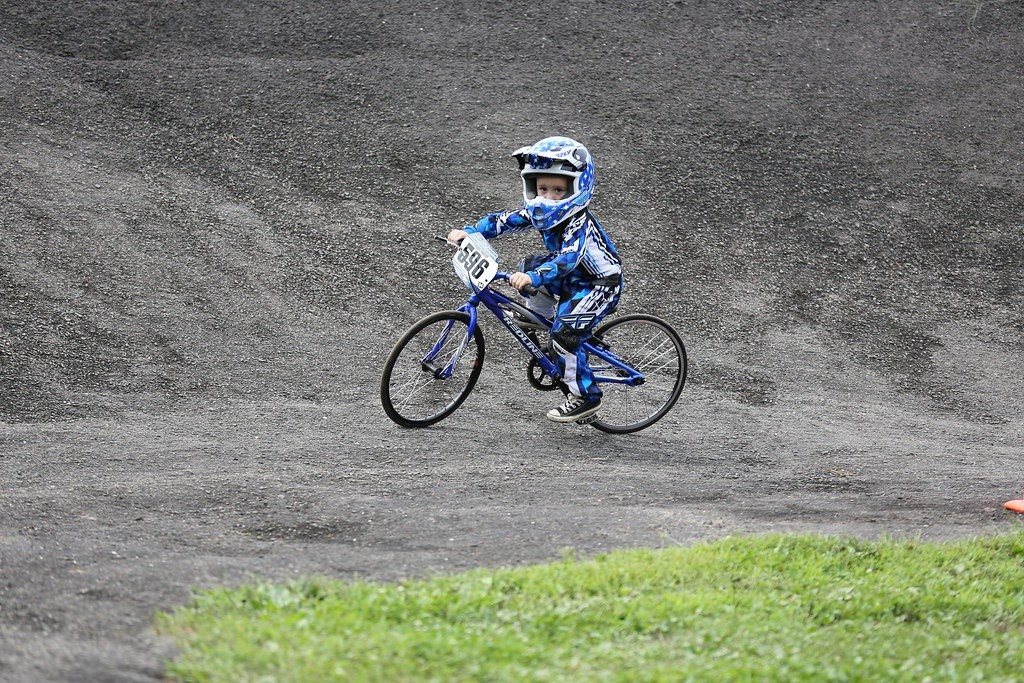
[504,310,554,330]
[546,393,602,423]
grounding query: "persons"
[448,137,624,423]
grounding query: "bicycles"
[378,233,688,435]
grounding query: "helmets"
[511,136,594,231]
